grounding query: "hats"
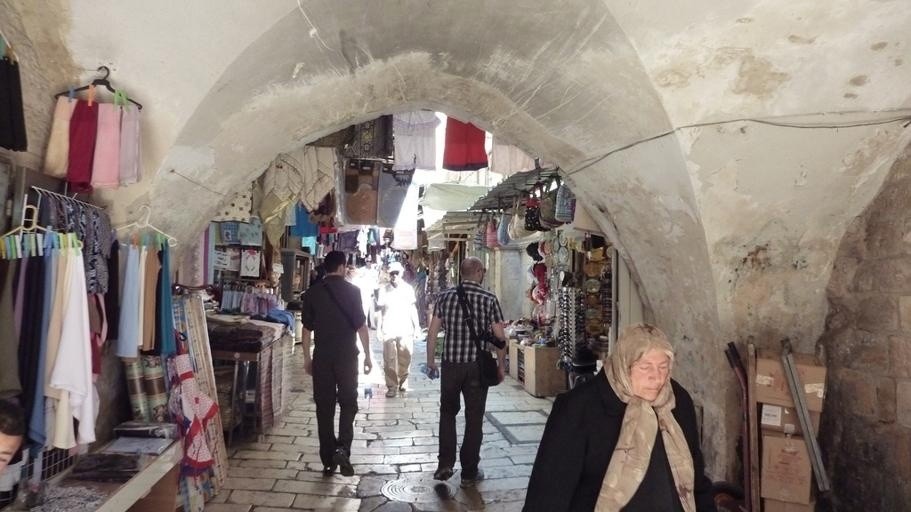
[526,239,553,305]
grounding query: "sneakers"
[434,467,484,488]
[324,447,354,476]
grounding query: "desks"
[213,345,272,447]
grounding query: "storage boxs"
[755,348,827,512]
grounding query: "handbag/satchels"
[473,175,576,250]
[477,350,505,386]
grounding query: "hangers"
[113,205,178,249]
[221,280,280,295]
[56,65,143,110]
[0,206,83,250]
[0,28,20,62]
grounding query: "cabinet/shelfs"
[281,248,311,303]
[509,339,567,396]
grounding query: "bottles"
[419,364,441,385]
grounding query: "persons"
[519,324,717,512]
[302,250,371,476]
[426,257,507,491]
[368,261,418,399]
[0,401,26,477]
